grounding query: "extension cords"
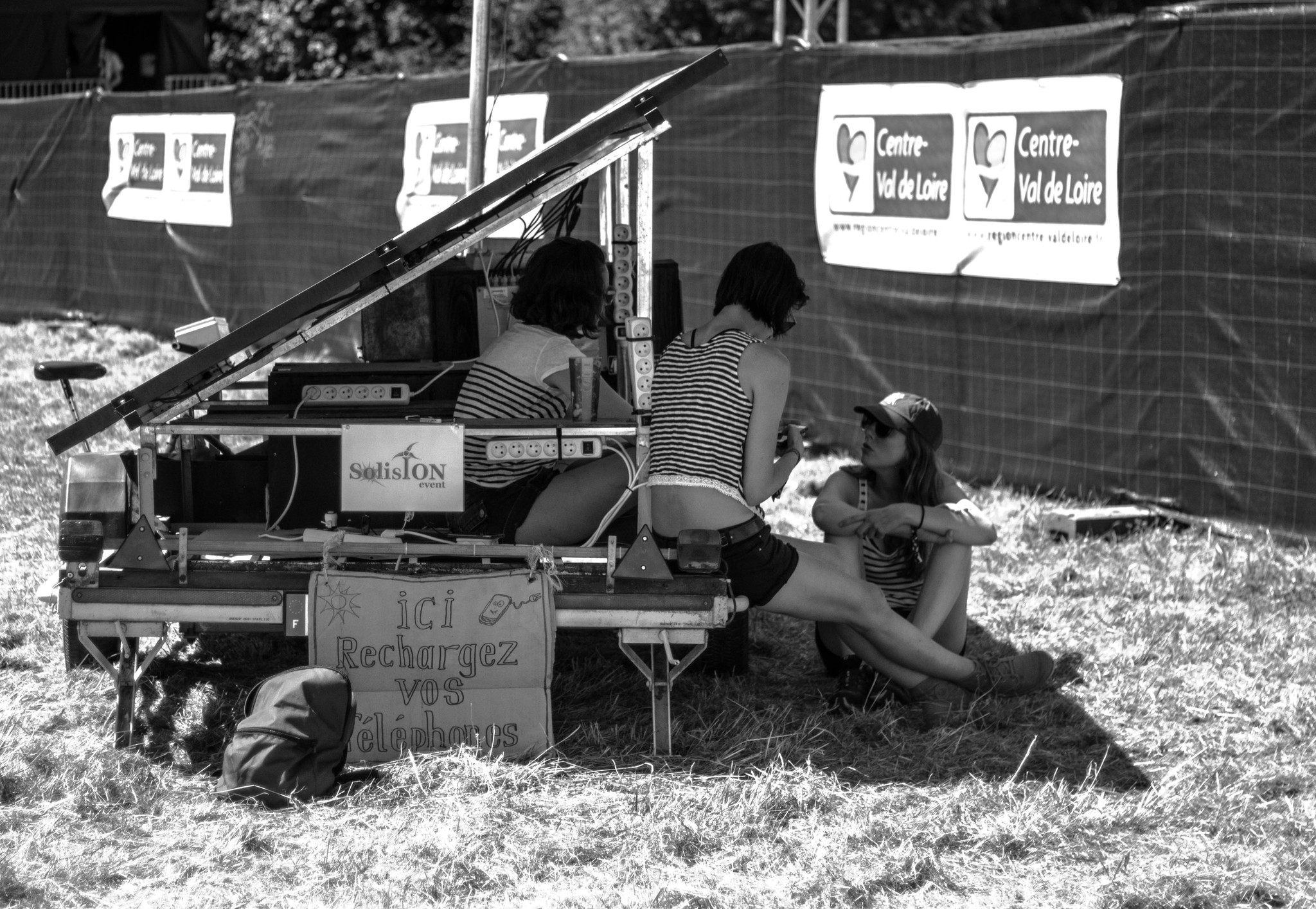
[626,316,655,413]
[485,437,603,461]
[302,383,410,407]
[612,224,635,347]
[303,527,404,543]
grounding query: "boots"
[956,651,1053,700]
[908,677,999,725]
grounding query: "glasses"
[782,307,796,332]
[861,412,894,439]
[602,289,615,305]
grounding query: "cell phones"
[777,424,808,447]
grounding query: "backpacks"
[212,664,379,808]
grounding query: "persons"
[647,242,1053,697]
[451,238,639,544]
[812,389,997,704]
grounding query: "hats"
[854,392,944,451]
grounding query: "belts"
[722,516,765,547]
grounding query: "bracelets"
[910,530,923,563]
[785,448,801,464]
[910,502,925,528]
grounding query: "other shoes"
[825,656,875,713]
[870,674,907,710]
